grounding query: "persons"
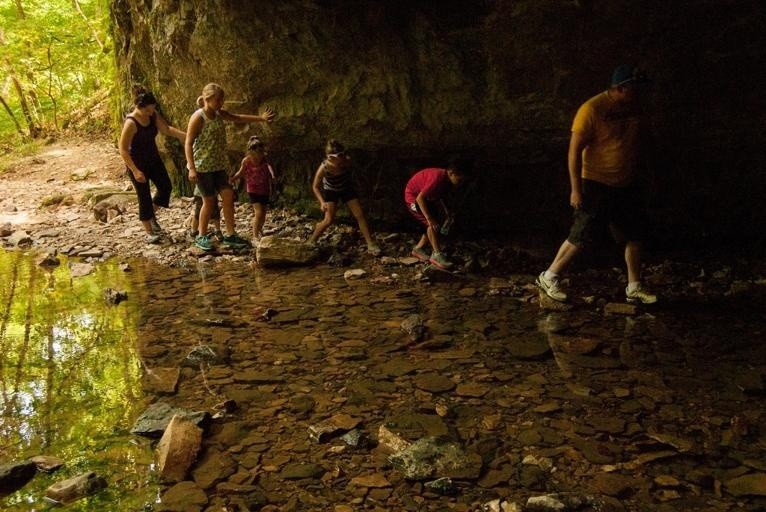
[189,183,224,242]
[307,139,382,258]
[118,93,187,243]
[534,62,663,307]
[183,82,276,252]
[193,267,237,397]
[403,161,467,269]
[544,312,670,411]
[246,260,277,317]
[227,135,276,249]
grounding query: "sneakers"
[144,215,263,252]
[429,252,453,269]
[534,269,568,302]
[625,284,658,305]
[411,244,430,261]
